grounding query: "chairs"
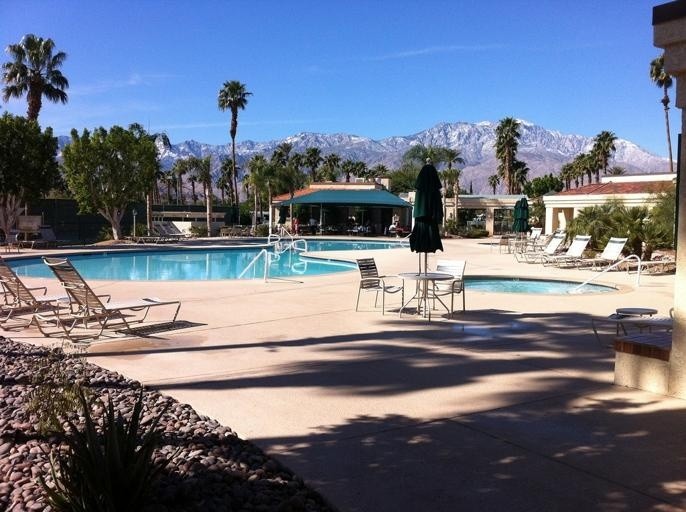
[491,227,629,271]
[151,220,186,246]
[0,223,71,250]
[0,252,181,342]
[354,257,467,325]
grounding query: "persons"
[308,216,318,235]
[387,222,397,232]
[292,214,299,233]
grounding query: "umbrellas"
[515,197,532,240]
[512,200,521,233]
[409,156,444,275]
